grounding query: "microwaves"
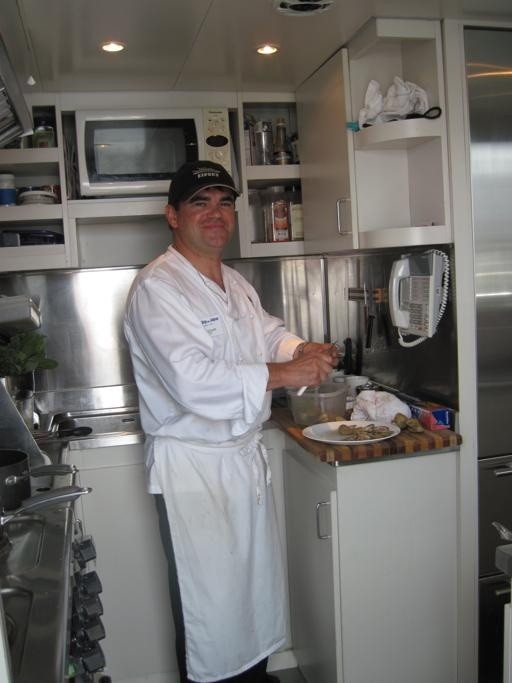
[75,107,230,197]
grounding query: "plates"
[303,421,401,444]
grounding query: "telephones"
[388,250,445,339]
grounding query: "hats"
[169,161,240,203]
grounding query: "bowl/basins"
[288,383,347,426]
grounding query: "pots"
[1,448,93,542]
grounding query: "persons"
[124,161,338,683]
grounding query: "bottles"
[14,389,34,438]
[254,117,292,165]
[0,174,17,206]
[248,184,303,244]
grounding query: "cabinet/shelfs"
[69,428,460,681]
[0,16,454,275]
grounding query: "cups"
[345,375,368,395]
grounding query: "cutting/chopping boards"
[270,406,462,463]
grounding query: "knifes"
[365,264,378,350]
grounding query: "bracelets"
[298,341,310,359]
